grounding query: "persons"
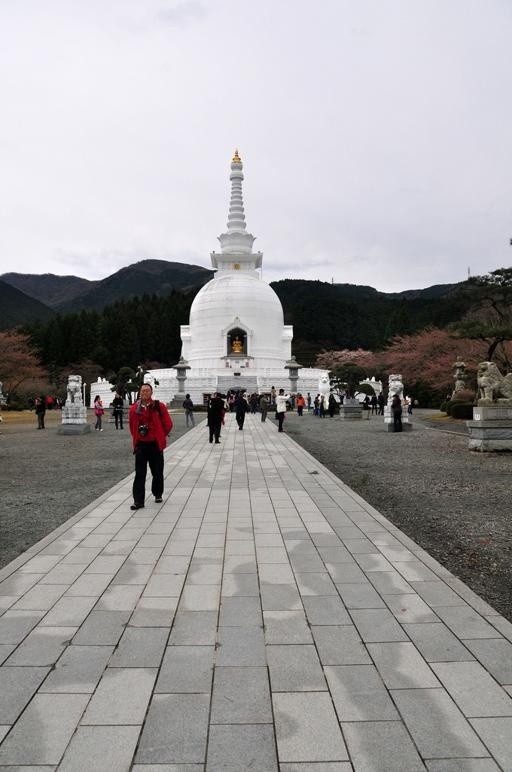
[111,393,124,430]
[405,394,413,415]
[232,336,243,352]
[364,392,385,416]
[182,394,196,428]
[450,355,469,375]
[93,395,105,432]
[27,388,64,430]
[204,385,336,444]
[128,384,173,510]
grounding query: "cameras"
[138,425,148,436]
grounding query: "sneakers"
[156,496,163,504]
[131,502,145,510]
